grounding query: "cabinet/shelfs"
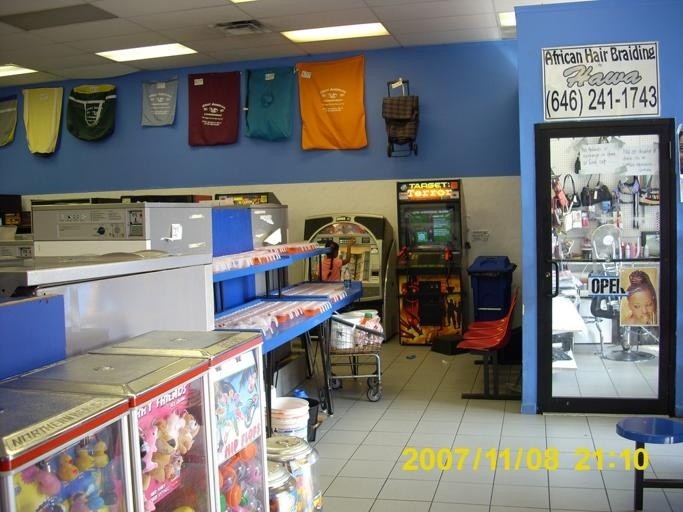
[88,330,270,512]
[0,384,135,512]
[213,245,364,439]
[549,261,660,344]
[0,352,217,512]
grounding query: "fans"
[591,225,623,261]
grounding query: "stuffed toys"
[139,403,207,512]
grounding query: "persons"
[624,270,657,326]
[318,237,355,283]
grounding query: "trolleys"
[382,79,419,158]
[319,311,383,401]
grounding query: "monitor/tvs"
[400,203,461,252]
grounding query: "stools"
[616,417,683,511]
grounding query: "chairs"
[591,296,654,363]
[457,288,522,400]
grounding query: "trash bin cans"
[467,256,517,321]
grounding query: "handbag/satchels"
[582,172,610,207]
[556,175,579,209]
[638,181,660,206]
[617,166,639,195]
[573,152,581,174]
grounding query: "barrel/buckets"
[272,396,309,441]
[303,397,320,441]
